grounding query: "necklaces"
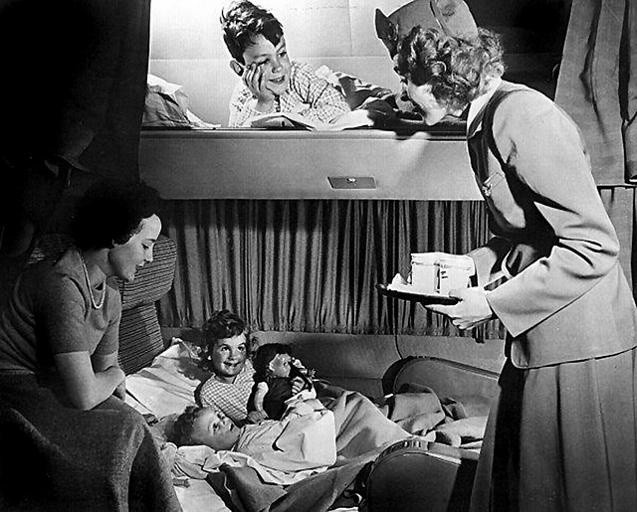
[82,258,107,309]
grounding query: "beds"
[118,123,507,512]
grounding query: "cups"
[440,254,475,295]
[411,252,440,293]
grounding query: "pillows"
[141,74,222,130]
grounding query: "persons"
[198,308,305,426]
[0,172,184,511]
[217,0,352,128]
[172,404,241,451]
[372,0,637,512]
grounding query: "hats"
[375,1,481,60]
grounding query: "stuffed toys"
[253,341,317,421]
